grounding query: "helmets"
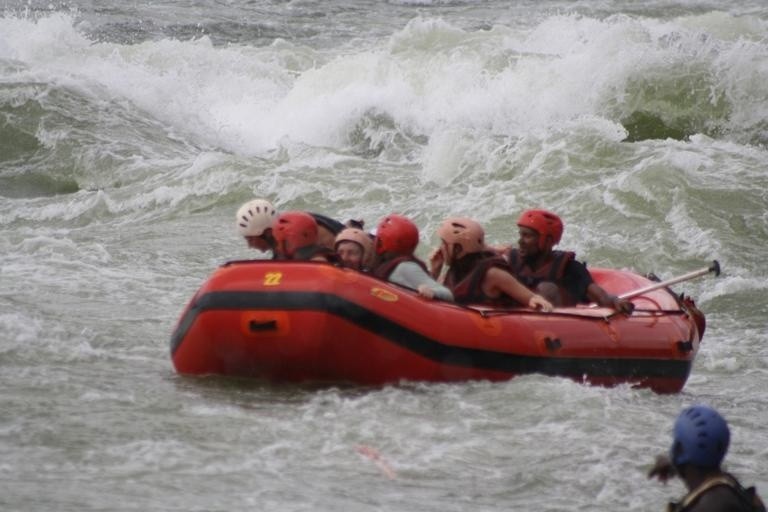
[236,199,276,236]
[273,212,320,255]
[373,214,419,255]
[438,218,485,263]
[669,405,732,468]
[516,210,563,247]
[334,229,370,264]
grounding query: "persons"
[369,212,457,304]
[271,210,335,266]
[427,216,555,317]
[333,228,373,272]
[647,402,767,512]
[497,207,636,318]
[232,199,375,266]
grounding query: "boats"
[163,253,717,395]
